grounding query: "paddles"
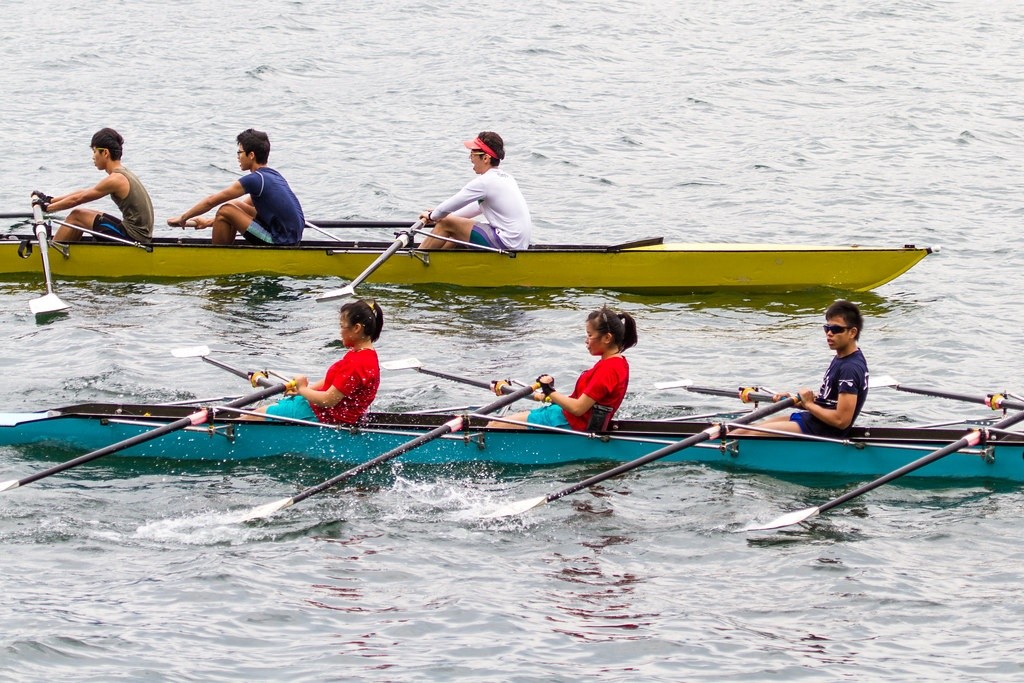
[169,345,297,396]
[731,409,1024,534]
[315,209,434,303]
[0,379,297,493]
[168,219,437,229]
[651,378,788,403]
[478,393,802,519]
[0,212,69,220]
[380,357,553,403]
[241,382,542,521]
[868,375,1024,410]
[28,194,74,317]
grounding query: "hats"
[464,137,501,160]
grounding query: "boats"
[0,403,1024,483]
[0,234,941,293]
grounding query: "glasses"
[237,150,246,156]
[823,324,857,334]
[471,151,485,158]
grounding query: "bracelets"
[428,212,435,221]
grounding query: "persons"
[486,302,638,432]
[167,129,305,245]
[417,130,531,250]
[234,298,383,425]
[30,128,154,241]
[728,300,869,434]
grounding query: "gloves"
[31,198,50,212]
[31,190,54,204]
